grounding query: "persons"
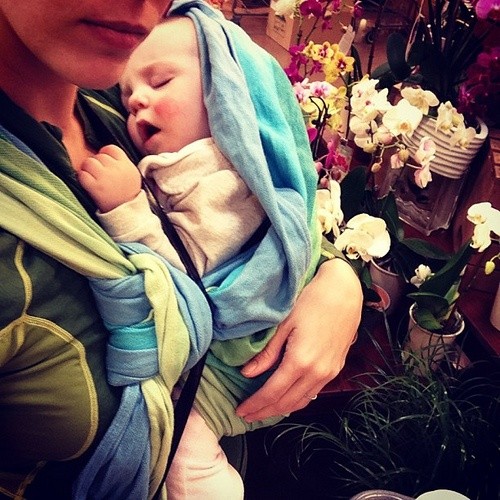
[78,0,319,500]
[0,0,364,500]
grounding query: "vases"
[490,286,500,334]
[370,256,404,315]
[409,303,465,361]
[405,116,489,180]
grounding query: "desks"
[316,223,500,396]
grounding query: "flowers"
[268,0,500,283]
[405,199,500,334]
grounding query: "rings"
[304,393,317,400]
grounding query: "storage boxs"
[266,0,355,53]
